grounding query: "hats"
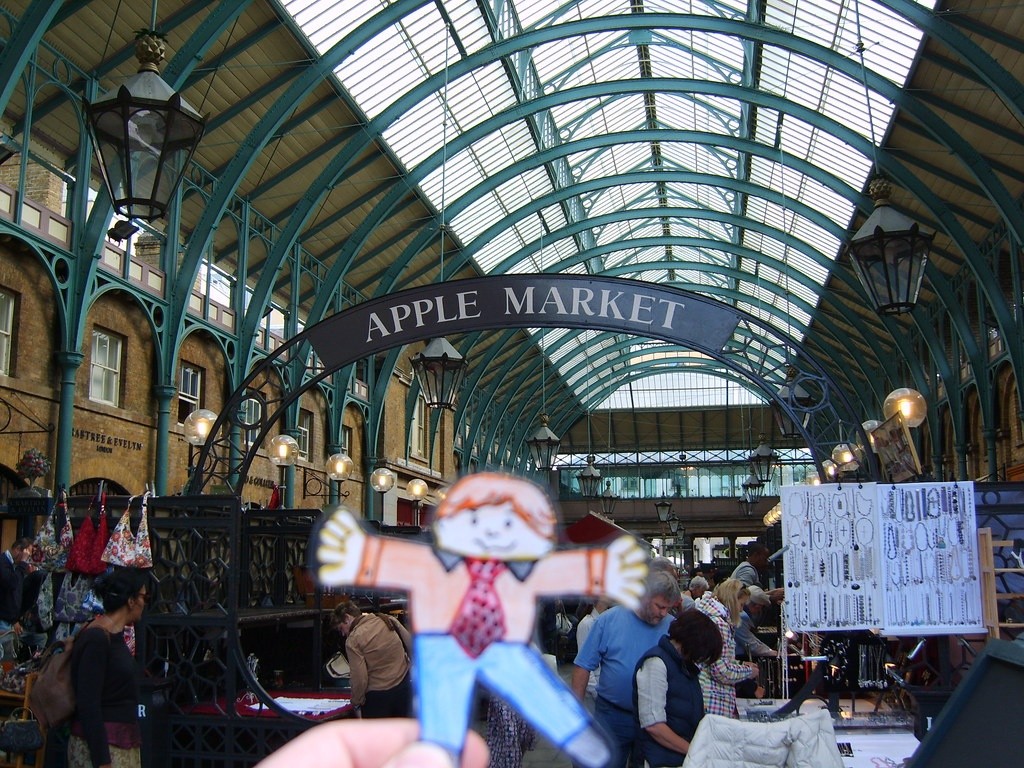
[748,585,770,606]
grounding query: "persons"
[570,571,677,768]
[330,601,412,719]
[67,567,149,768]
[734,585,778,699]
[685,579,759,720]
[729,543,769,589]
[576,601,609,703]
[632,608,723,768]
[0,537,33,625]
[683,576,710,601]
[253,718,490,768]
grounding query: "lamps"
[742,389,764,503]
[524,215,562,472]
[79,0,214,223]
[844,0,937,316]
[852,417,884,456]
[770,122,816,439]
[677,523,686,576]
[667,508,679,564]
[762,500,783,527]
[600,364,619,514]
[410,22,473,409]
[736,493,754,516]
[830,441,862,476]
[747,260,780,485]
[183,409,453,526]
[654,491,673,560]
[575,326,603,499]
[820,459,836,485]
[808,473,821,485]
[882,387,928,430]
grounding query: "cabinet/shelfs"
[980,525,1024,641]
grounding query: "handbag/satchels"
[0,707,44,752]
[25,488,154,660]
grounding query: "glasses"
[138,593,152,603]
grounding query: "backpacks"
[27,618,110,728]
